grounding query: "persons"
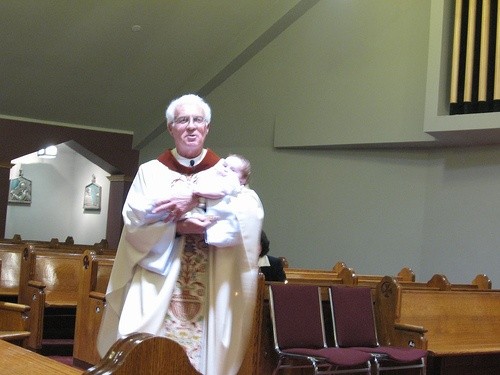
[128,153,252,225]
[258,230,286,283]
[97,93,264,374]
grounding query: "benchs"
[0,234,117,370]
[0,332,203,375]
[237,258,500,375]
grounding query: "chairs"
[268,284,373,375]
[328,286,427,375]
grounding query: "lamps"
[37,145,57,159]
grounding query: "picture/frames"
[83,182,102,211]
[7,177,32,204]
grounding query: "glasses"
[173,116,208,124]
